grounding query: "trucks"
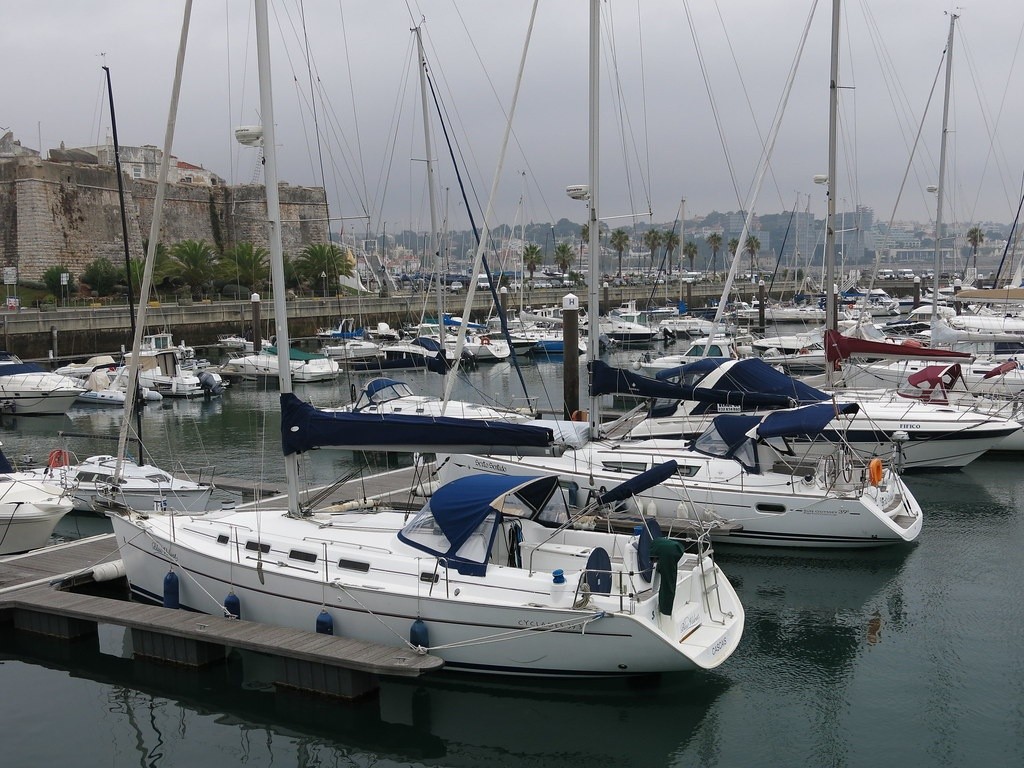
[478,274,493,290]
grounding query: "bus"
[678,272,702,284]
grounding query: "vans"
[921,269,935,279]
[877,269,894,280]
[897,268,915,280]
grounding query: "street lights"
[366,223,370,256]
[383,221,387,263]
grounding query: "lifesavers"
[49,449,70,467]
[869,459,883,485]
[481,337,491,346]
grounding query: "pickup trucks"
[412,282,449,292]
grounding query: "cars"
[645,277,665,285]
[950,273,962,279]
[550,280,562,288]
[563,279,575,287]
[450,281,462,294]
[509,280,528,290]
[939,272,949,278]
[627,278,642,287]
[534,280,553,289]
[613,279,626,288]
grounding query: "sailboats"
[0,0,1024,680]
